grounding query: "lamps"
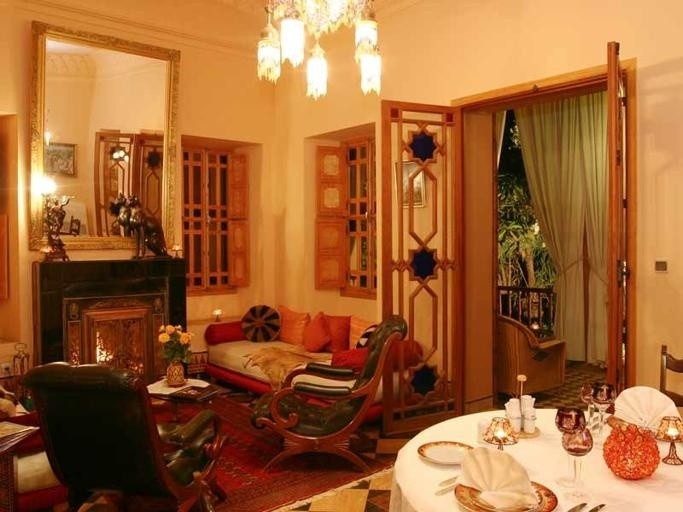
[253,0,384,102]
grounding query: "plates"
[416,440,475,466]
[454,480,557,512]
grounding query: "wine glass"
[560,426,592,498]
[554,402,587,488]
[580,381,616,448]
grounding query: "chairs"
[26,360,230,512]
[248,313,408,474]
[495,315,565,399]
[659,343,683,407]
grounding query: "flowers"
[157,323,194,360]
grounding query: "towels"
[453,445,539,509]
[613,385,682,437]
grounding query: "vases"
[166,357,185,386]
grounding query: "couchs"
[203,303,424,423]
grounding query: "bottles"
[11,341,29,405]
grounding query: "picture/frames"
[43,142,76,177]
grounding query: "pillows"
[348,315,376,348]
[273,305,309,344]
[302,310,332,353]
[325,314,349,357]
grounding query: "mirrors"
[29,19,181,246]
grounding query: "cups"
[503,393,537,433]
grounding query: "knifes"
[567,503,587,512]
[589,503,606,511]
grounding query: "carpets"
[0,377,399,512]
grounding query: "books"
[170,386,220,401]
